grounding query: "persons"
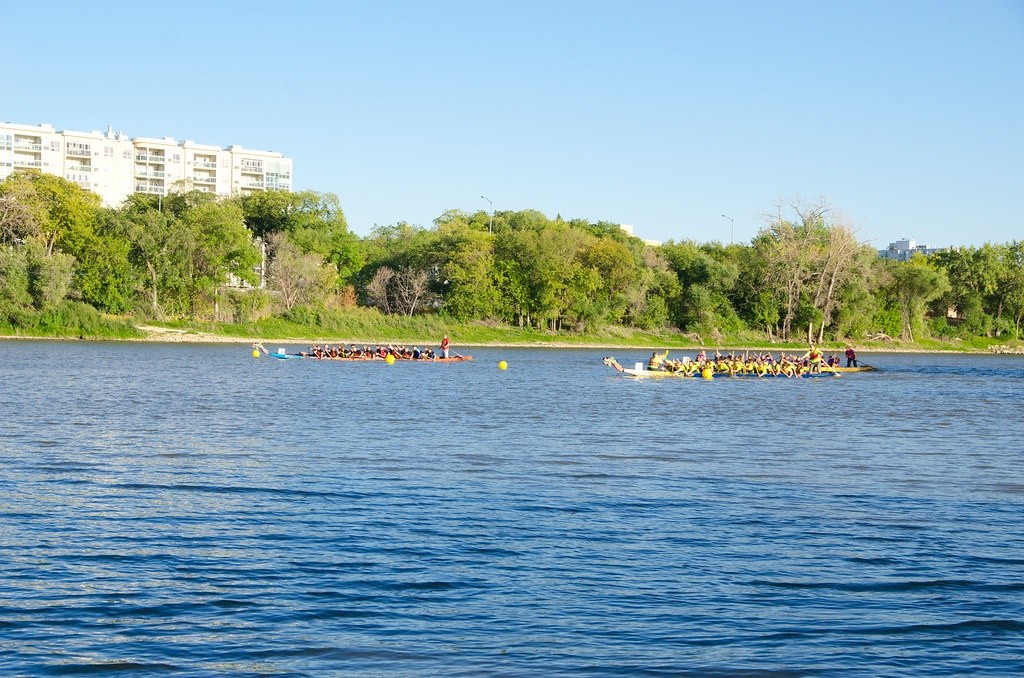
[845,345,857,367]
[439,335,449,359]
[647,351,666,371]
[800,344,824,374]
[310,343,437,360]
[667,349,840,378]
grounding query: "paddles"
[449,349,464,359]
[846,356,886,372]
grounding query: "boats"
[603,356,875,378]
[252,342,472,363]
